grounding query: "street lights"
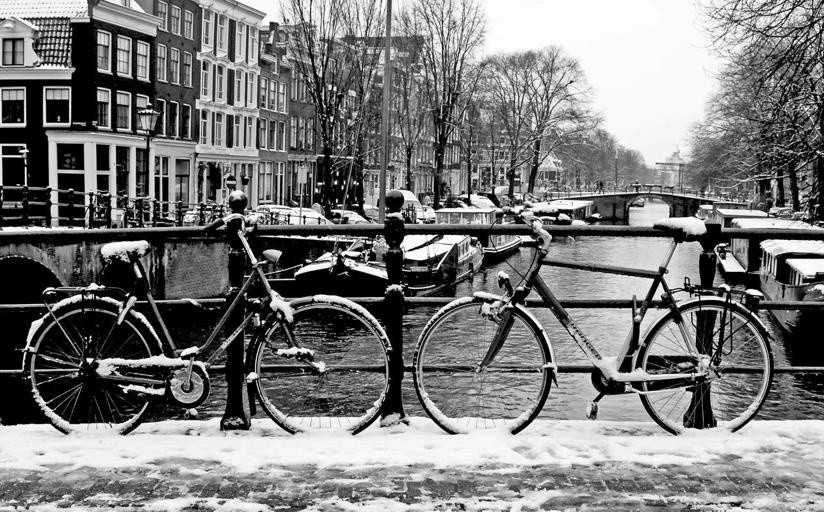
[137,97,163,227]
[614,156,620,186]
[563,166,569,192]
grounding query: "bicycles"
[12,187,398,443]
[411,214,778,438]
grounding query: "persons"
[424,192,431,205]
[594,180,604,195]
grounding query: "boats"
[693,191,824,343]
[530,192,598,239]
[433,204,526,261]
[632,199,645,207]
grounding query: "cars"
[544,183,594,193]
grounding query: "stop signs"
[225,176,236,190]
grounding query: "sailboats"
[283,1,492,290]
